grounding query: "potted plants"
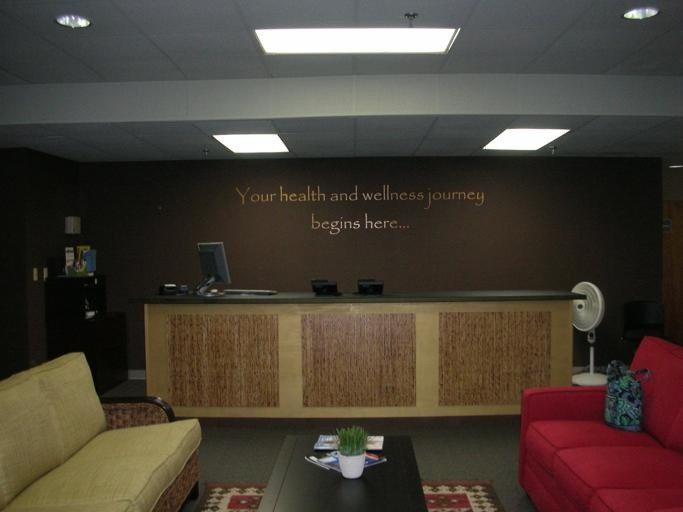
[334,425,367,481]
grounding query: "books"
[65,245,96,273]
[304,435,387,473]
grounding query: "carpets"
[192,480,505,512]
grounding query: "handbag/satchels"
[604,360,652,432]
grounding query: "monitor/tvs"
[193,242,232,297]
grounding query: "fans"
[570,279,609,385]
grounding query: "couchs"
[516,337,683,512]
[0,350,203,512]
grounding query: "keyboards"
[224,289,278,295]
